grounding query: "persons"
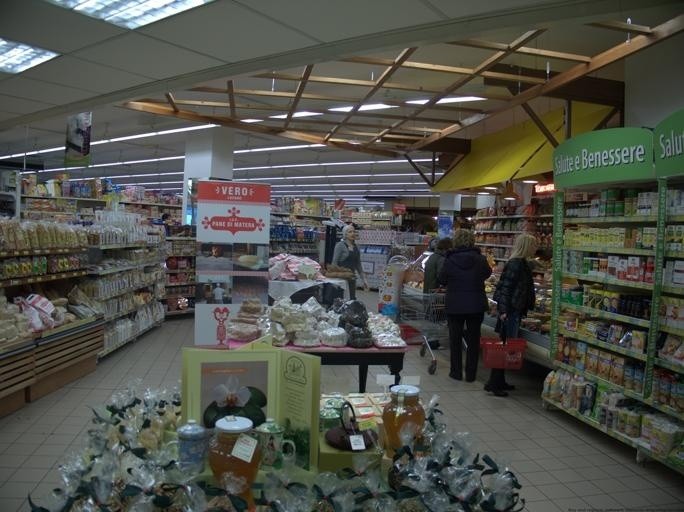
[422,237,453,350]
[484,233,538,398]
[161,213,172,236]
[438,228,493,382]
[332,224,369,300]
[212,282,226,303]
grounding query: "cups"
[256,418,296,468]
[166,419,208,473]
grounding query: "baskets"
[480,337,527,371]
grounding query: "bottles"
[381,385,427,460]
[206,415,261,494]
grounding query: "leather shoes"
[484,381,515,397]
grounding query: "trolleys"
[397,284,468,374]
